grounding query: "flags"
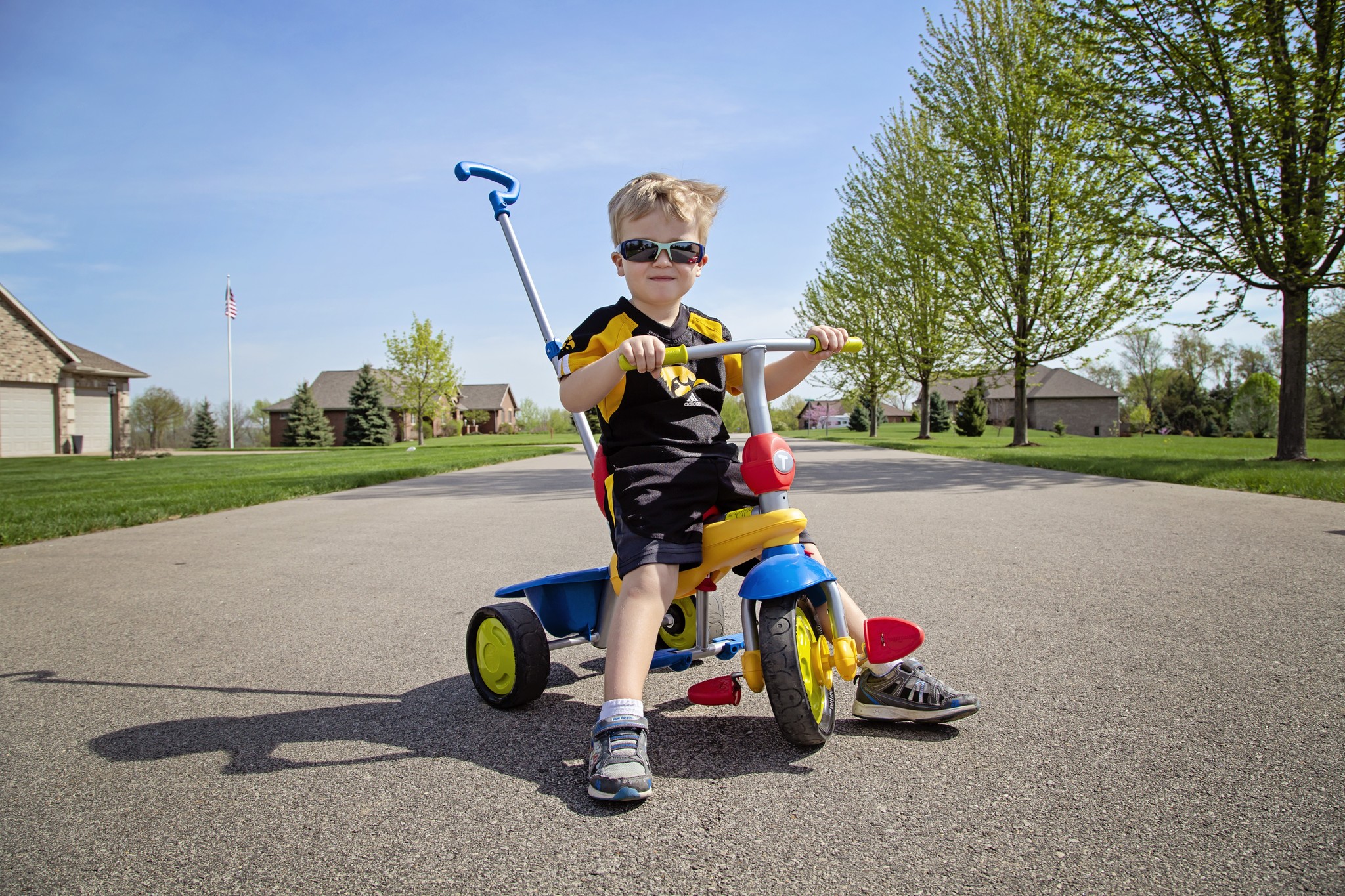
[224,284,237,320]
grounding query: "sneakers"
[588,714,652,802]
[852,658,980,724]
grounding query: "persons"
[560,173,981,801]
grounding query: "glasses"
[615,239,706,264]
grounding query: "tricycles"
[454,163,924,754]
